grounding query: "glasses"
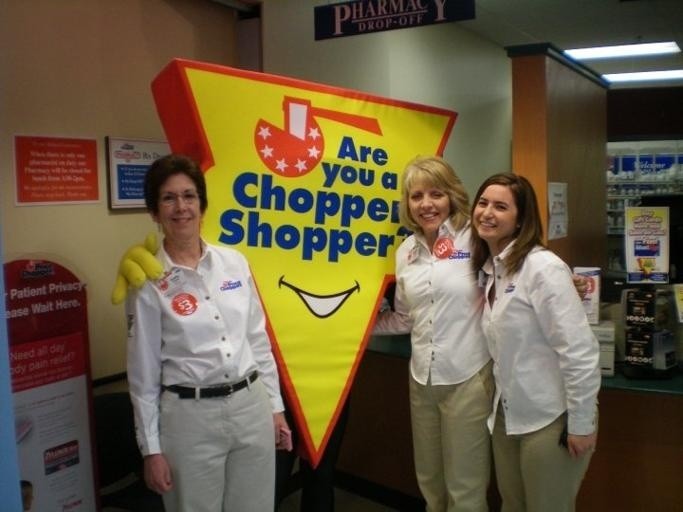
[157,191,199,204]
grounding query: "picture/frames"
[105,135,172,211]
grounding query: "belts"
[161,370,258,398]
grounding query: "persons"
[124,155,290,511]
[370,157,588,511]
[472,173,602,511]
